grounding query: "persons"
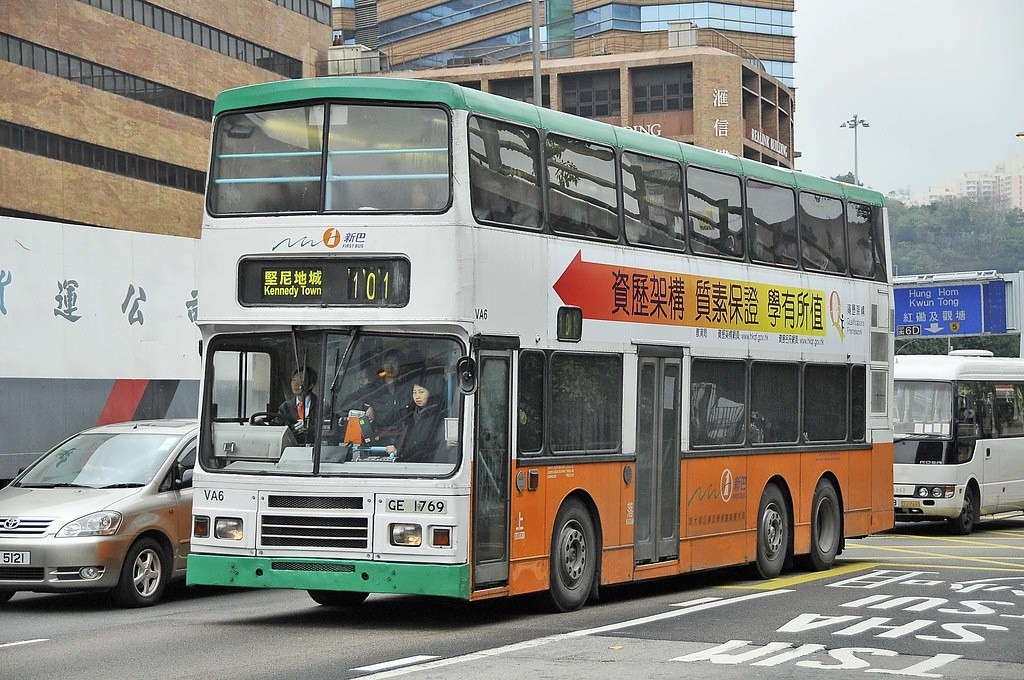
[337,348,411,455]
[254,175,302,212]
[270,366,330,444]
[407,179,431,209]
[385,377,448,463]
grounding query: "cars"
[0,418,200,608]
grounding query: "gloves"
[295,420,305,431]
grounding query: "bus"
[185,77,895,613]
[893,349,1024,534]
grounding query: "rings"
[388,450,390,452]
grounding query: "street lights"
[839,113,870,186]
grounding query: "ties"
[298,401,304,419]
